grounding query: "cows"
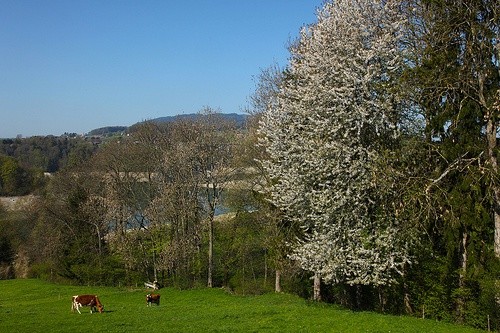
[71,295,104,315]
[145,293,161,307]
